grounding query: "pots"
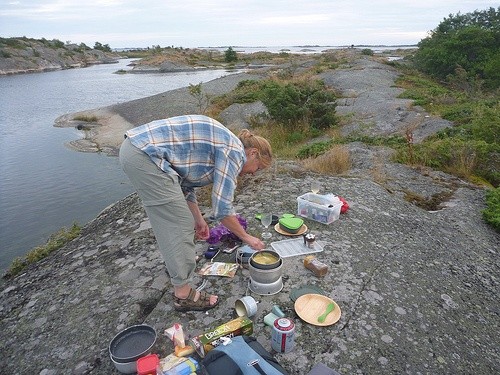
[252,249,282,269]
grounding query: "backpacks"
[200,334,289,375]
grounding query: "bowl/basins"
[268,215,279,225]
[235,296,258,318]
[110,352,150,374]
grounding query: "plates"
[109,324,157,363]
[274,223,308,236]
[294,294,341,326]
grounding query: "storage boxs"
[296,192,344,226]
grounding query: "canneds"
[304,256,328,276]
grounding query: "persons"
[120,114,272,311]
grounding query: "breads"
[175,345,196,357]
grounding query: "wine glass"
[261,212,272,239]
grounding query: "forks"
[318,303,334,322]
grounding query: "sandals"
[164,255,202,277]
[172,287,219,312]
